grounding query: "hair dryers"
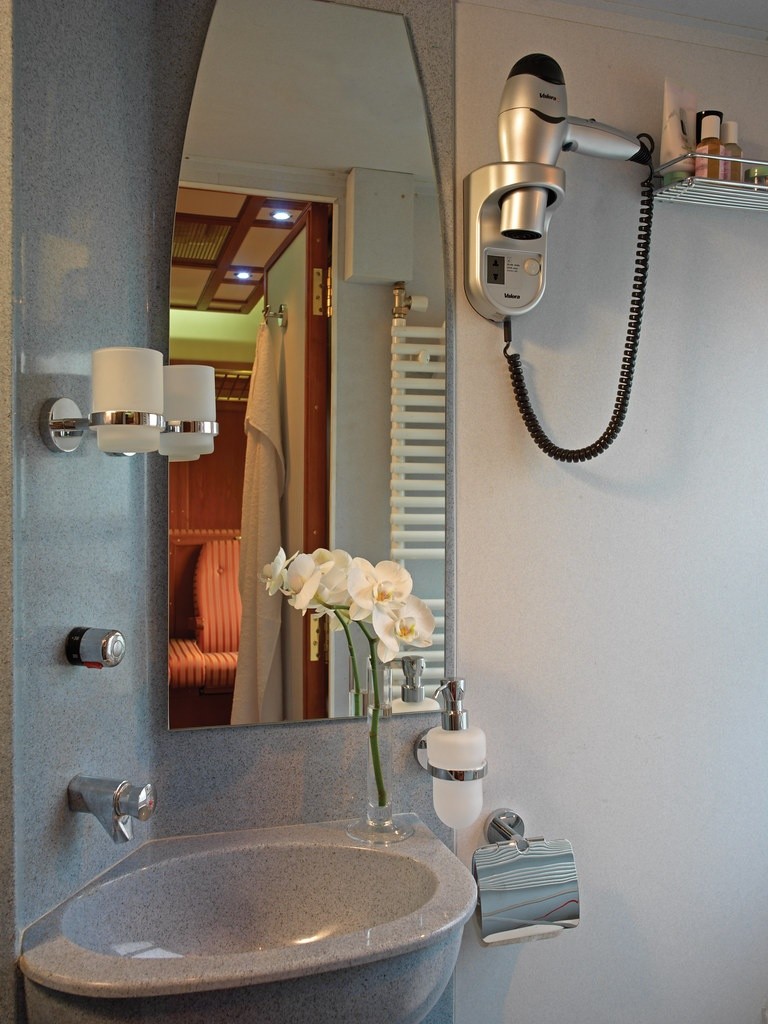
[493,51,653,241]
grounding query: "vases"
[343,655,417,846]
[348,654,374,719]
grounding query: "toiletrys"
[748,165,768,187]
[692,109,726,147]
[374,651,443,716]
[425,676,490,833]
[692,115,725,183]
[718,121,748,191]
[660,76,699,191]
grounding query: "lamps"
[40,342,218,466]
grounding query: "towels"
[225,320,288,728]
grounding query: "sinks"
[15,806,480,1024]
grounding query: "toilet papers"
[470,894,570,951]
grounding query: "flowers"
[280,546,430,806]
[255,546,362,718]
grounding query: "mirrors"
[165,16,448,731]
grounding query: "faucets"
[65,773,151,850]
